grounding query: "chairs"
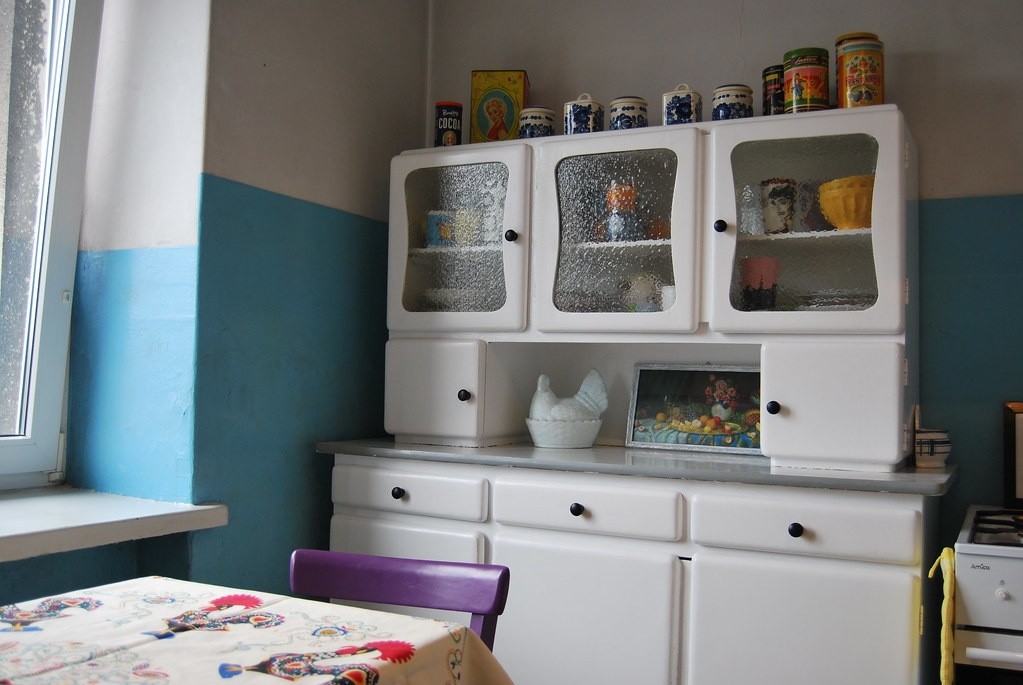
[290,549,510,652]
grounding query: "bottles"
[761,64,784,117]
[915,429,951,468]
[711,84,753,121]
[434,101,463,147]
[609,95,647,128]
[839,39,885,107]
[835,32,879,107]
[518,106,555,138]
[606,176,639,240]
[783,47,829,113]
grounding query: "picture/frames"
[624,362,766,455]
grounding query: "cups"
[661,285,676,310]
[759,176,812,234]
[661,83,703,125]
[562,93,604,134]
[739,255,777,312]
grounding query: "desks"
[0,576,514,685]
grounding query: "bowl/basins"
[818,174,874,230]
[526,418,602,448]
[426,288,492,309]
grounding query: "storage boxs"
[470,69,530,143]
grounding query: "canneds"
[763,31,884,115]
[434,100,462,145]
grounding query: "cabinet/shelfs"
[314,434,960,684]
[315,105,922,475]
